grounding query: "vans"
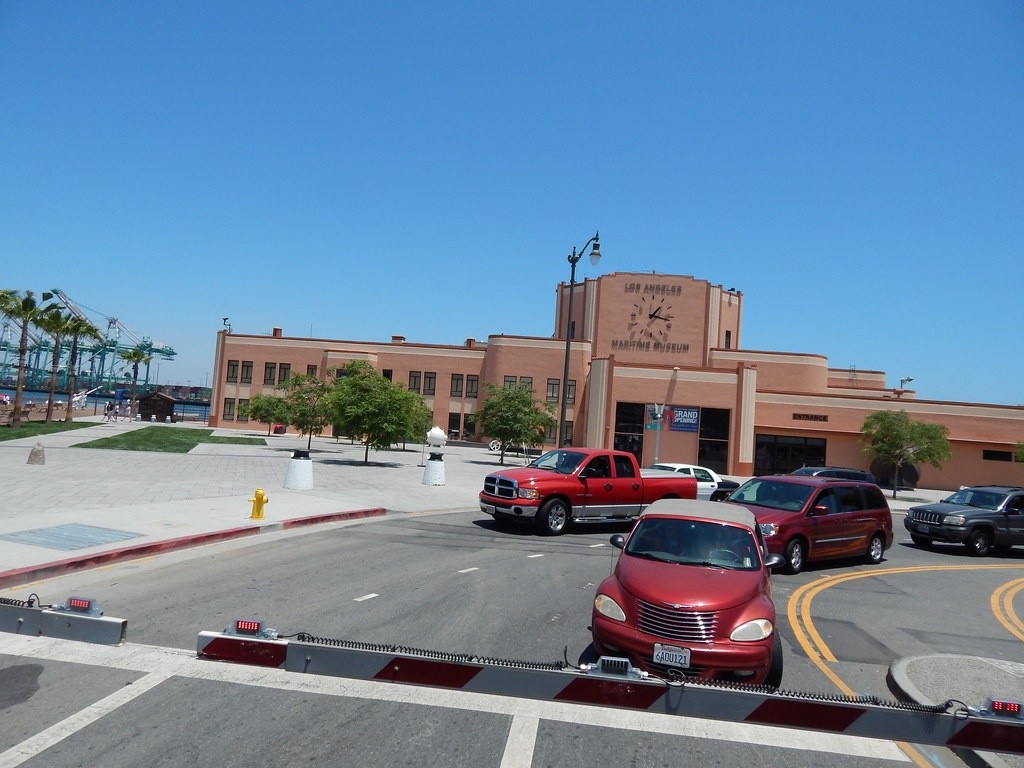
[719,473,896,575]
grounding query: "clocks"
[630,292,675,339]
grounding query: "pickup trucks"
[478,446,698,535]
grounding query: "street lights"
[554,230,601,467]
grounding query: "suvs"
[790,466,875,489]
[903,485,1024,558]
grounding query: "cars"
[648,462,745,502]
[585,497,784,693]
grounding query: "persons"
[1,392,88,411]
[640,521,686,559]
[101,401,132,423]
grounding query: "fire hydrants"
[247,488,268,520]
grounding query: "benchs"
[4,410,31,423]
[23,404,37,411]
[52,403,63,410]
